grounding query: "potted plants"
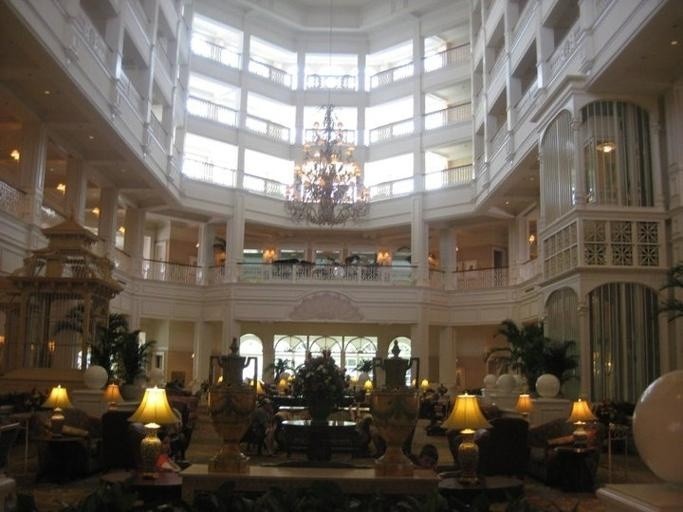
[493,320,582,397]
[80,314,158,400]
[289,350,354,422]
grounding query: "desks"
[440,477,523,511]
[180,462,440,512]
[28,434,80,483]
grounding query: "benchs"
[274,408,376,457]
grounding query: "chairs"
[523,413,606,492]
[85,409,190,480]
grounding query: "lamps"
[39,380,181,485]
[514,394,597,448]
[439,392,494,485]
[282,89,374,226]
